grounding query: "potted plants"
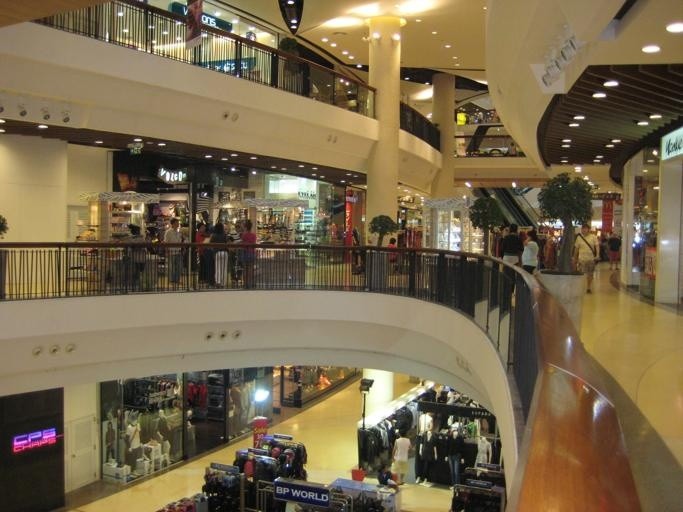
[537,172,595,340]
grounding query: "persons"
[392,430,413,487]
[127,414,143,468]
[128,213,260,287]
[239,381,251,434]
[574,225,601,295]
[378,463,387,484]
[607,233,620,270]
[271,447,283,457]
[474,437,494,477]
[388,238,406,262]
[443,430,466,488]
[106,422,117,462]
[522,230,541,279]
[415,430,439,484]
[155,409,173,443]
[500,222,524,278]
[386,472,398,489]
